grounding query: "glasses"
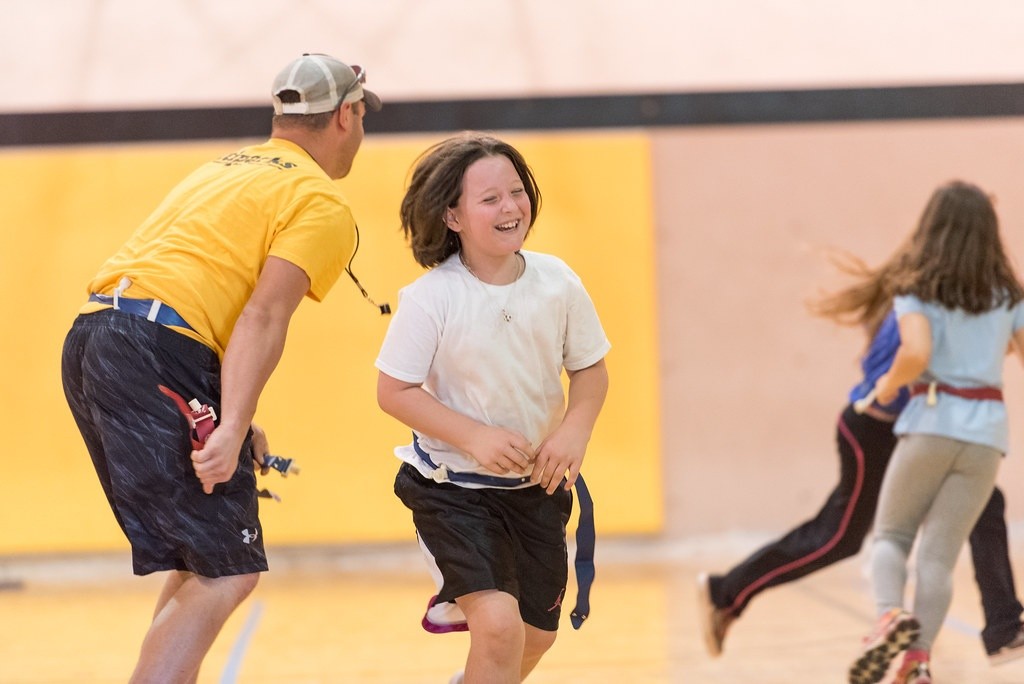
[335,66,366,110]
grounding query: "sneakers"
[698,574,739,657]
[988,623,1024,664]
[892,650,931,684]
[848,608,921,684]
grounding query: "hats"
[271,53,382,115]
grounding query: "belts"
[87,292,193,331]
[910,382,1003,400]
[412,431,595,630]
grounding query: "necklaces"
[459,251,522,323]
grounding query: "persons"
[57,52,383,684]
[808,182,1024,684]
[376,135,611,683]
[700,258,1024,664]
